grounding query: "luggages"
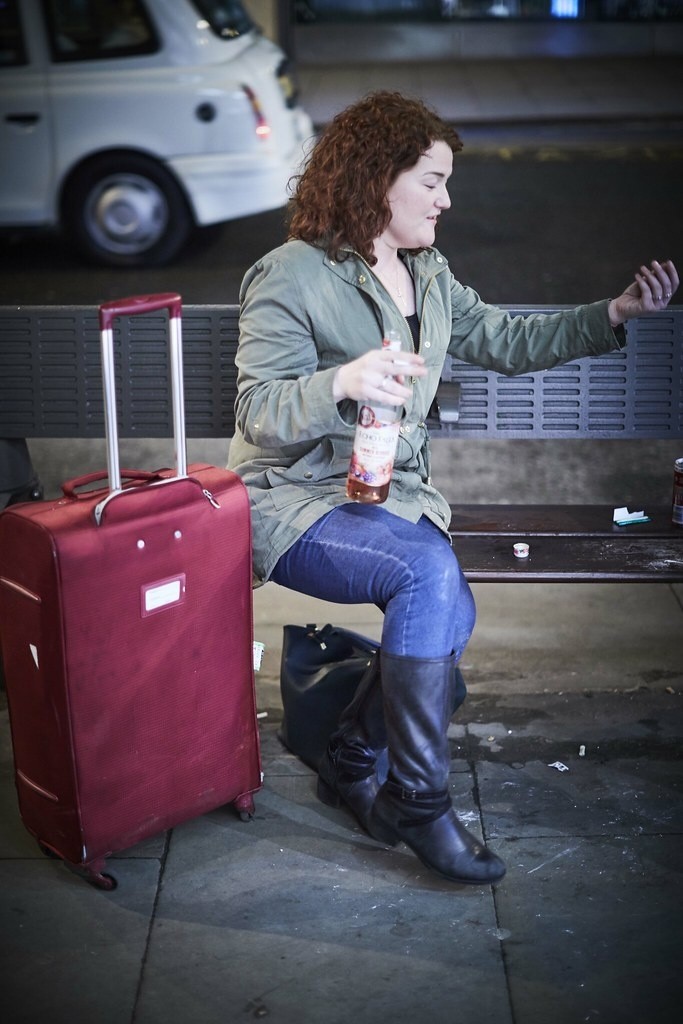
[0,290,265,891]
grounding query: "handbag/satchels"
[276,623,467,785]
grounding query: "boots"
[366,647,509,886]
[312,649,401,848]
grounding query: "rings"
[663,292,672,298]
[652,296,663,300]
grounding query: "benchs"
[0,303,683,586]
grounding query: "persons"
[226,90,679,885]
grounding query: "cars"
[1,0,325,269]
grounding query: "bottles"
[347,332,404,505]
